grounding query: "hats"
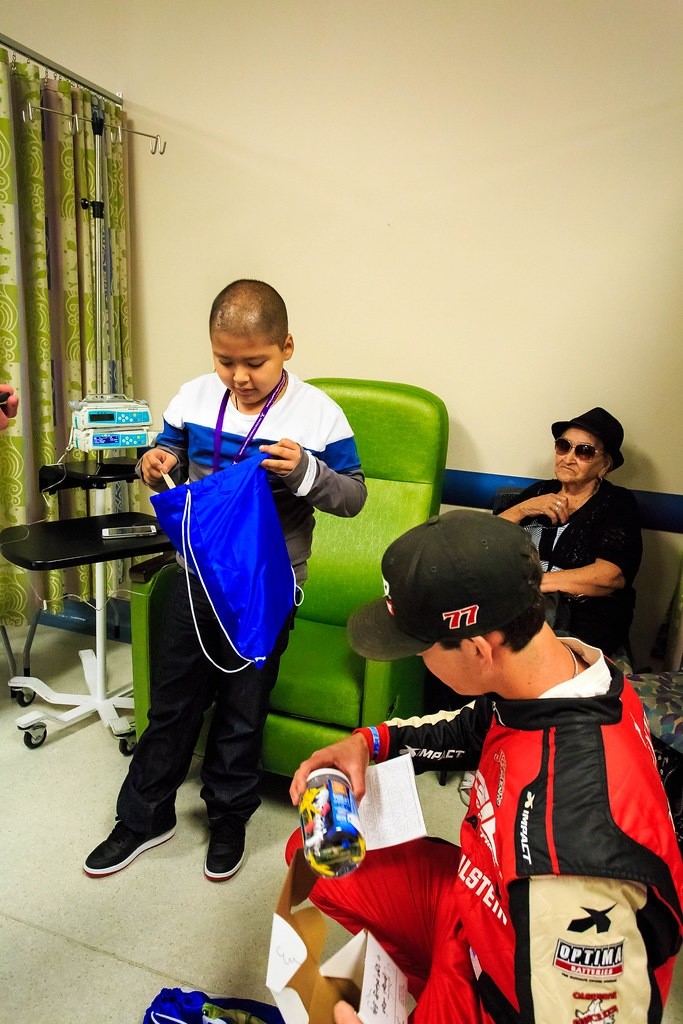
[551,407,625,472]
[345,508,541,661]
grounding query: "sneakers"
[205,818,245,881]
[84,812,178,877]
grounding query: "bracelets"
[366,726,380,760]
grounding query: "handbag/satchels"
[148,450,306,673]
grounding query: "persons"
[0,383,19,432]
[457,406,644,807]
[82,277,368,883]
[288,508,683,1024]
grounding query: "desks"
[0,511,173,757]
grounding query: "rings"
[556,500,562,507]
[555,507,559,513]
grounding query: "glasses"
[554,437,604,463]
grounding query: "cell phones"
[101,526,157,539]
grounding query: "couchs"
[129,377,450,780]
